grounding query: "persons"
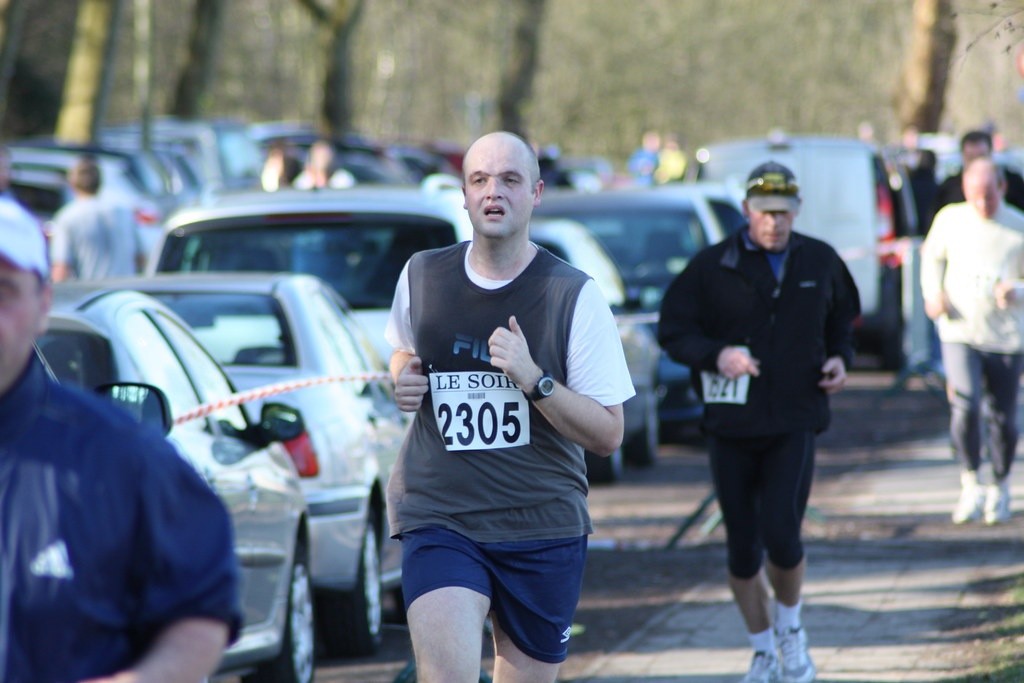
[0,200,244,683]
[632,131,688,186]
[48,161,148,281]
[656,163,861,683]
[289,141,353,191]
[895,124,1024,524]
[386,131,638,683]
[539,149,576,188]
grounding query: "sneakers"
[772,620,816,683]
[740,651,781,683]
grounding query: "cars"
[116,269,408,654]
[10,114,753,489]
[28,288,319,683]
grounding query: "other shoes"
[985,482,1010,525]
[952,485,983,524]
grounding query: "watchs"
[521,370,555,402]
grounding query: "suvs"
[688,134,931,376]
[159,173,477,414]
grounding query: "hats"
[745,160,803,214]
[0,196,48,279]
